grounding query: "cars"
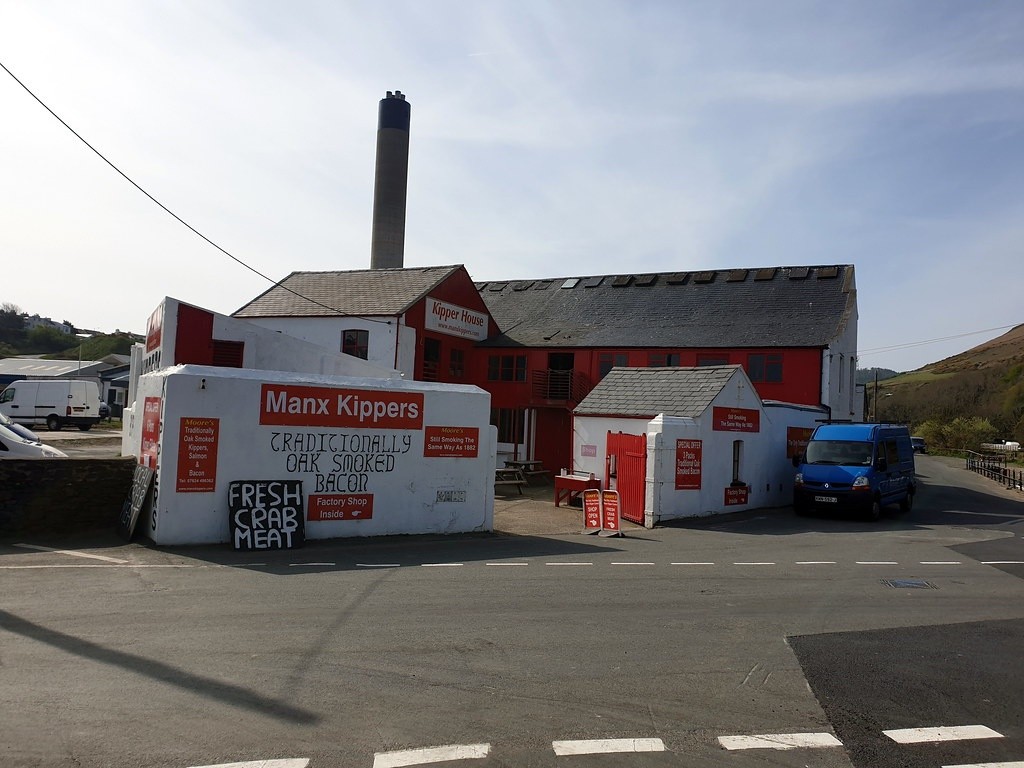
[0,409,69,458]
[98,396,109,419]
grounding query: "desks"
[503,460,552,487]
[495,468,528,495]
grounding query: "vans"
[793,424,918,522]
[910,437,927,454]
[0,380,101,431]
